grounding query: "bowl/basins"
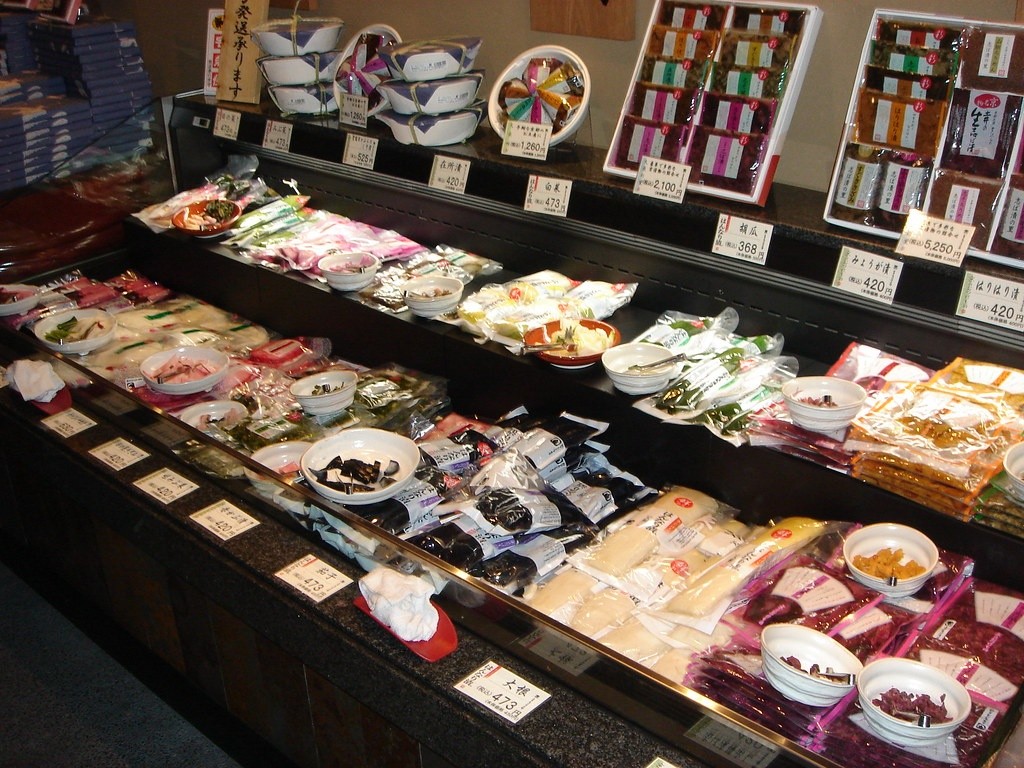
[254,17,487,147]
[1003,442,1024,500]
[760,624,863,707]
[842,523,938,598]
[399,276,463,318]
[783,376,867,432]
[488,45,591,149]
[0,285,43,317]
[301,428,421,504]
[173,200,241,237]
[244,442,312,501]
[35,308,117,355]
[317,252,379,291]
[523,317,620,369]
[857,658,971,746]
[139,348,229,395]
[602,342,676,395]
[291,369,358,415]
[180,400,248,432]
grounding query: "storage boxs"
[602,0,1024,273]
[0,0,157,192]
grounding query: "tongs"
[522,343,577,355]
[627,353,686,373]
[158,364,190,383]
[338,263,365,274]
[409,291,431,298]
